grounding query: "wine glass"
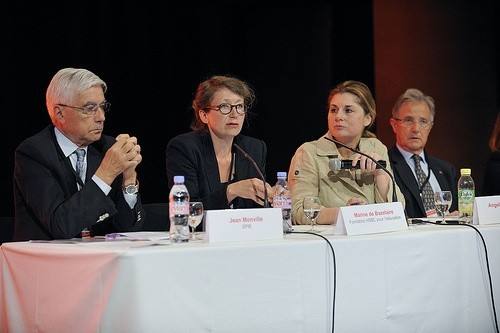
[302,197,322,233]
[188,201,203,241]
[434,191,452,224]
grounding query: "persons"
[387,88,480,218]
[166,76,291,232]
[287,81,406,225]
[13,67,146,242]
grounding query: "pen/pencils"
[436,220,466,224]
[233,174,240,181]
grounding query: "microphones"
[324,136,399,202]
[231,141,268,207]
[328,158,388,172]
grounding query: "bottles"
[457,169,475,224]
[273,172,292,229]
[169,176,190,243]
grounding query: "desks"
[0,224,500,333]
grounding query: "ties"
[73,149,86,191]
[411,154,435,212]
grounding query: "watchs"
[122,179,139,196]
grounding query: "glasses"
[58,102,111,116]
[205,102,247,115]
[396,117,434,129]
